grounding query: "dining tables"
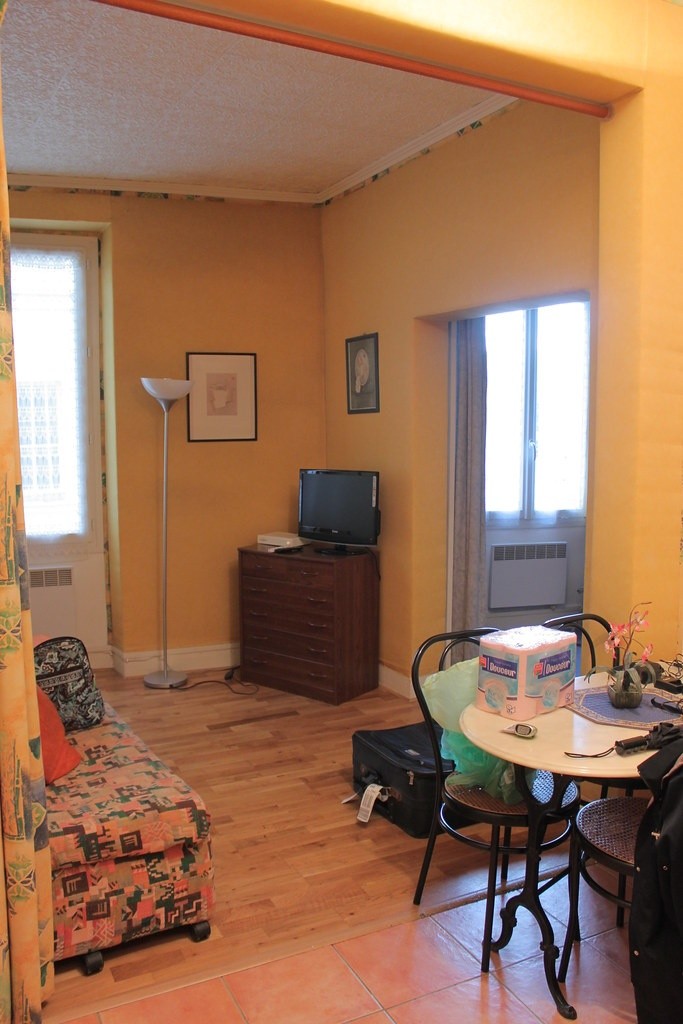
[457,673,683,1022]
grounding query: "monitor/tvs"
[298,468,380,555]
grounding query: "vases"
[608,669,642,708]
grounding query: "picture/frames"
[343,332,382,415]
[183,352,261,444]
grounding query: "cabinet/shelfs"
[236,534,380,708]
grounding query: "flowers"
[585,602,658,686]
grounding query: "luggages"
[350,719,480,837]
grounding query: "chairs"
[541,612,648,866]
[410,624,588,973]
[557,797,653,982]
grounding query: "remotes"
[275,546,303,554]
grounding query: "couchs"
[44,691,217,977]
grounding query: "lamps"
[137,375,196,691]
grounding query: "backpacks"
[35,636,102,730]
